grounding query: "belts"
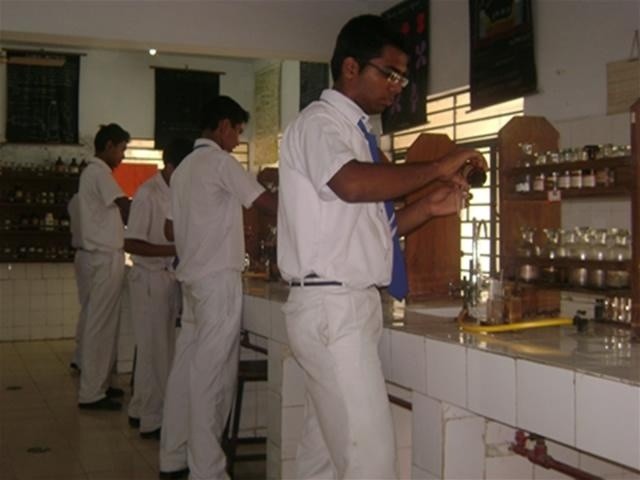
[291,280,342,286]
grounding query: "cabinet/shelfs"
[498,96,640,343]
[0,167,79,263]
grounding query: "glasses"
[365,60,409,88]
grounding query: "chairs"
[221,361,269,479]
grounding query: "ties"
[358,118,409,302]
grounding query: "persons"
[123,139,194,439]
[276,14,487,480]
[67,185,81,371]
[158,93,277,480]
[76,124,131,411]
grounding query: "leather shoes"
[78,387,125,410]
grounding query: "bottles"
[461,161,485,186]
[457,184,465,218]
[256,238,271,281]
[1,155,90,256]
[515,141,629,188]
[514,224,634,282]
